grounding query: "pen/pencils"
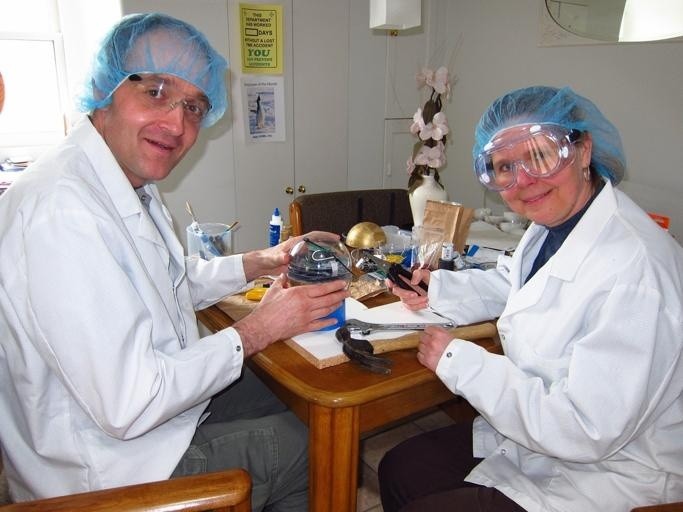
[190,222,225,262]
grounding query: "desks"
[187,238,504,512]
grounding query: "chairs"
[284,189,415,237]
[0,469,253,512]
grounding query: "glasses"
[127,75,213,127]
[475,126,582,193]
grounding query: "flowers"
[413,67,450,175]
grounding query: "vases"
[409,173,448,232]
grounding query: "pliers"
[363,251,428,296]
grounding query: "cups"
[473,208,529,236]
[187,223,232,261]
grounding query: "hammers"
[336,323,497,376]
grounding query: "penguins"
[250,93,271,128]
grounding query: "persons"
[0,11,351,511]
[375,83,682,510]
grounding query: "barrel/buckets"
[281,235,353,333]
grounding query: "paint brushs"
[220,222,239,238]
[185,200,196,221]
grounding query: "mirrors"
[543,0,683,44]
